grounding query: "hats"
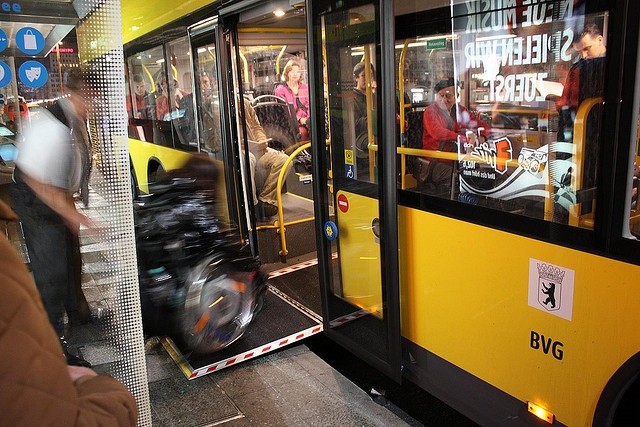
[433,79,454,95]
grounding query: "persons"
[157,71,192,119]
[0,199,138,426]
[204,67,293,220]
[353,63,379,179]
[154,45,177,78]
[423,80,487,198]
[276,60,311,129]
[180,72,212,141]
[553,22,606,160]
[517,115,541,147]
[126,74,149,120]
[14,68,112,366]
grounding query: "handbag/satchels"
[412,109,455,185]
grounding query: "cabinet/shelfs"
[251,94,306,167]
[274,80,286,88]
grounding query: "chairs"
[202,89,224,158]
[406,105,447,195]
[170,96,199,150]
[253,82,272,94]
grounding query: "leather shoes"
[255,201,278,216]
[254,208,270,223]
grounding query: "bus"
[41,0,638,426]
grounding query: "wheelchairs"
[132,176,268,356]
